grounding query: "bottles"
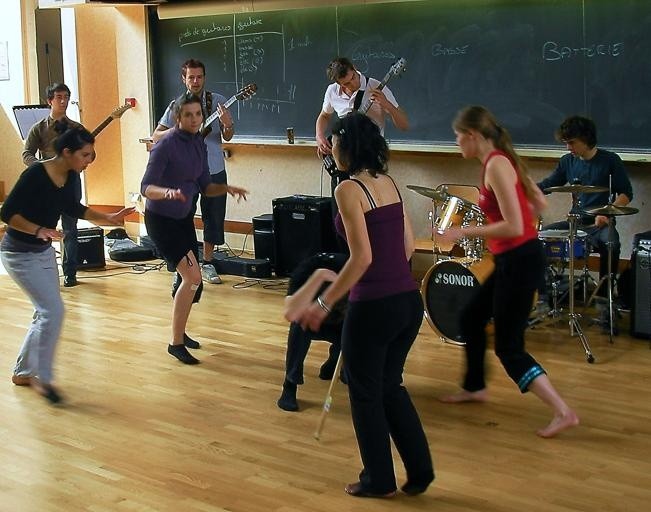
[286,128,296,144]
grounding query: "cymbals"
[587,205,639,215]
[406,185,451,202]
[544,186,609,192]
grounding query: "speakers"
[252,213,276,272]
[60,226,106,270]
[272,194,347,276]
[630,229,651,339]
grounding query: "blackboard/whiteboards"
[143,2,651,165]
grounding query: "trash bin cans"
[632,239,651,339]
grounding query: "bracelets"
[166,188,171,193]
[35,226,43,238]
[224,125,233,131]
[316,297,333,315]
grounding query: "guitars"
[323,57,409,178]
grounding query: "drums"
[537,230,588,257]
[433,196,484,244]
[420,253,496,346]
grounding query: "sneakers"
[200,264,221,284]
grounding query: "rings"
[371,100,373,102]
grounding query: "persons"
[436,105,580,437]
[23,81,96,289]
[0,119,95,402]
[316,58,407,198]
[154,59,235,285]
[301,113,434,499]
[278,252,348,411]
[141,97,249,365]
[537,116,633,306]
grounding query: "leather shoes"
[64,271,78,289]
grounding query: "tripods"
[586,247,623,346]
[527,249,568,325]
[549,253,596,364]
